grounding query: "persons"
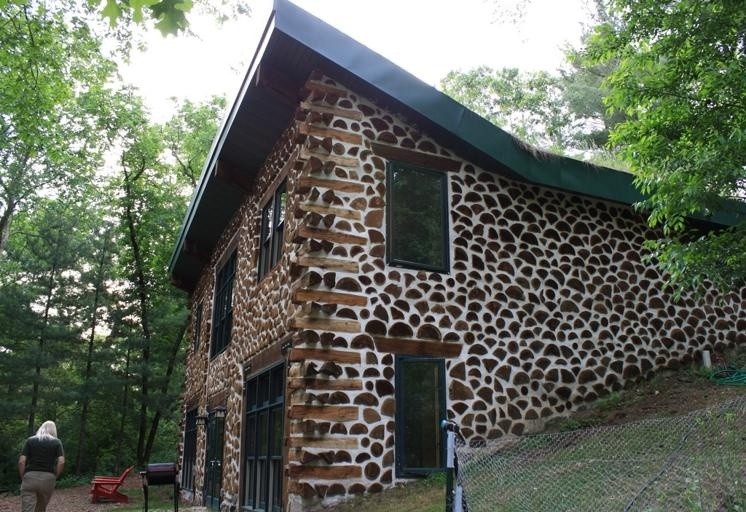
[17,419,66,512]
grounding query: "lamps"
[213,405,226,418]
[194,415,207,426]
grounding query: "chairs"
[91,466,136,503]
